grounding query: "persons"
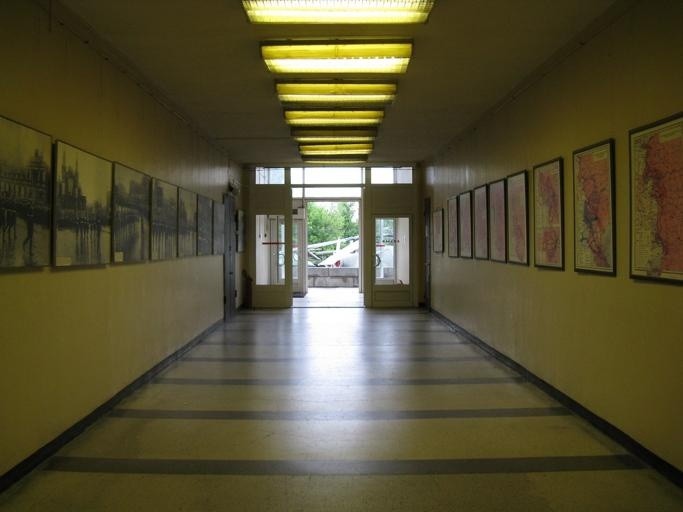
[0,190,102,257]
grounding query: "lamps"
[291,129,378,142]
[302,154,368,163]
[282,108,383,127]
[257,38,415,75]
[298,143,373,154]
[241,0,433,27]
[271,79,396,104]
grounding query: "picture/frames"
[178,184,247,257]
[111,159,149,265]
[628,112,683,283]
[151,178,177,261]
[0,115,52,271]
[572,137,619,275]
[55,139,112,268]
[506,168,530,266]
[532,155,565,269]
[431,177,505,262]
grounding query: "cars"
[278,252,315,267]
[316,239,392,267]
